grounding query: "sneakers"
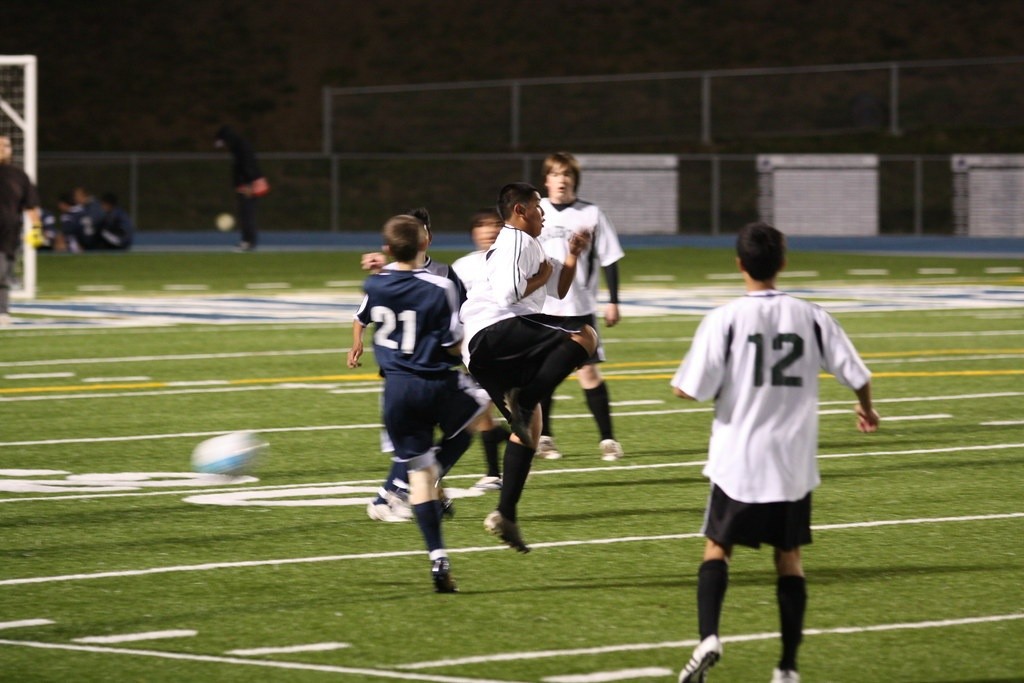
[432,480,456,517]
[504,386,536,448]
[679,633,722,683]
[432,557,457,594]
[483,509,529,556]
[771,668,799,683]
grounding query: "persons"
[668,222,878,683]
[0,134,39,323]
[34,187,137,257]
[345,152,626,592]
[215,127,269,249]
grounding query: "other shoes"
[367,479,417,522]
[535,437,562,459]
[599,437,622,461]
[469,474,504,494]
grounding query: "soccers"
[190,430,246,476]
